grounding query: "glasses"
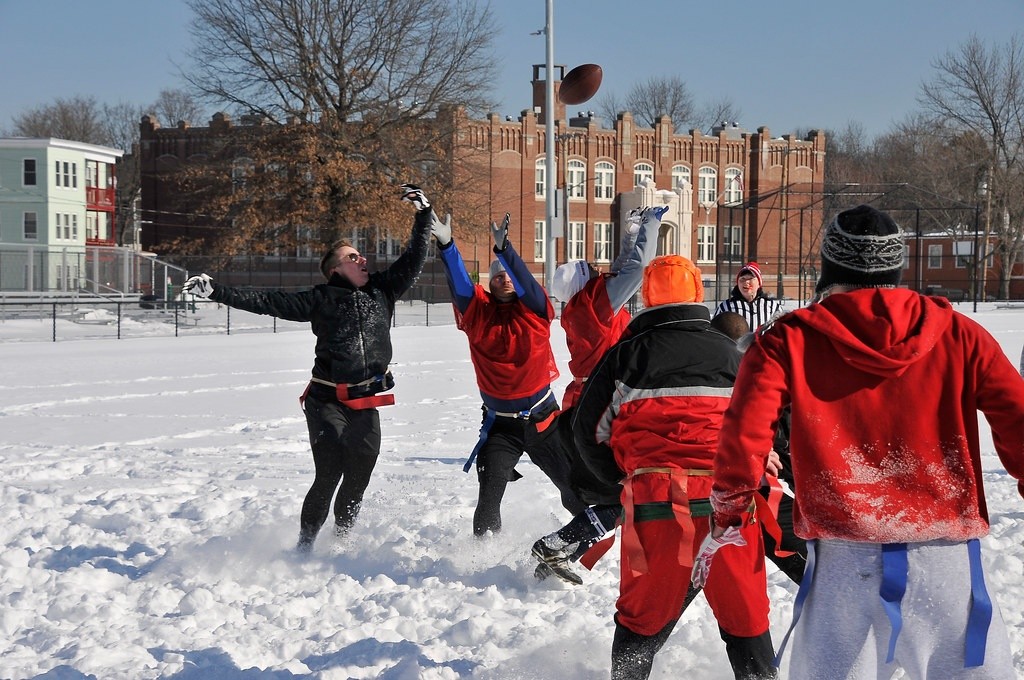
[326,252,363,273]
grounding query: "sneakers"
[531,539,583,585]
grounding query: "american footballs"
[558,64,603,105]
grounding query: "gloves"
[429,207,452,245]
[492,211,510,252]
[624,206,643,234]
[399,181,430,210]
[642,206,669,223]
[690,512,748,589]
[180,272,216,299]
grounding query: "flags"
[735,173,744,190]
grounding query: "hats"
[488,258,506,285]
[736,262,763,288]
[816,204,905,296]
[641,253,704,306]
[553,260,590,305]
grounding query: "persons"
[571,254,779,680]
[711,312,806,590]
[431,210,589,535]
[710,205,1024,680]
[710,262,782,331]
[531,205,665,585]
[180,184,432,554]
